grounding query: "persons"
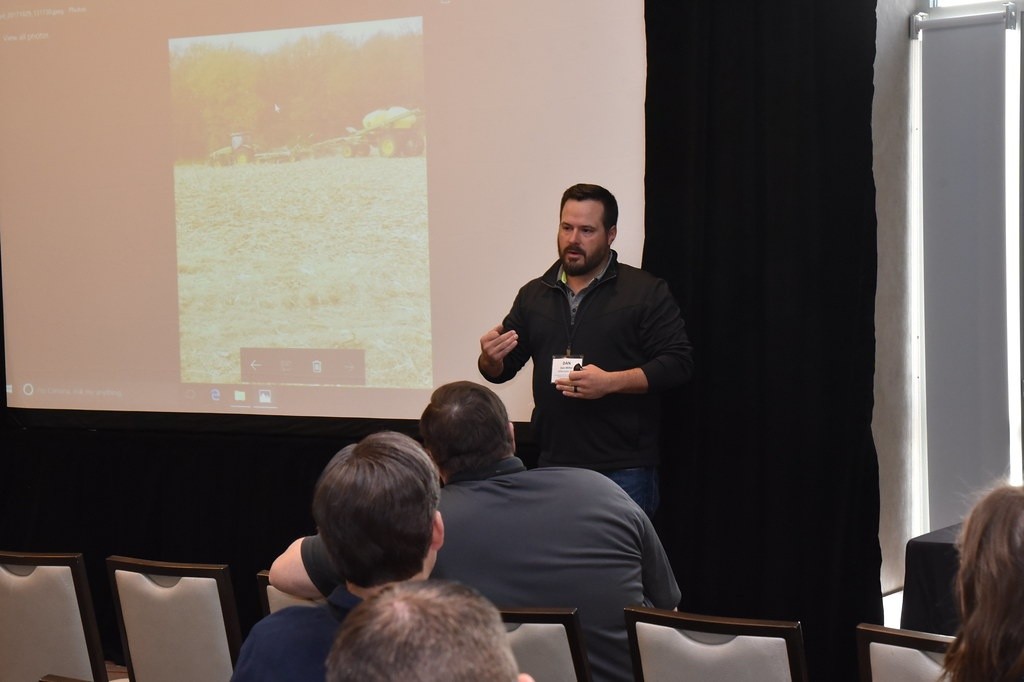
[230,431,445,682]
[938,486,1024,682]
[477,183,693,522]
[327,581,533,682]
[269,381,681,682]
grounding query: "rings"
[574,386,578,393]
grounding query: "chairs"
[0,549,959,682]
